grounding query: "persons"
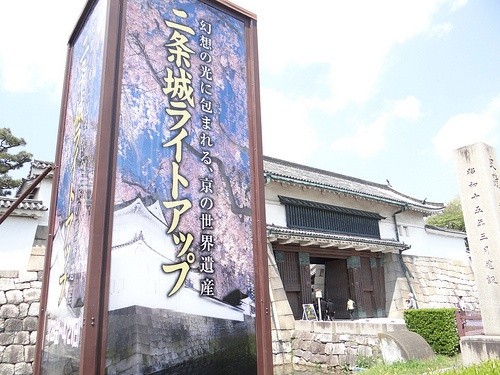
[407,293,417,310]
[347,297,357,321]
[405,299,412,310]
[456,294,466,316]
[313,297,336,321]
